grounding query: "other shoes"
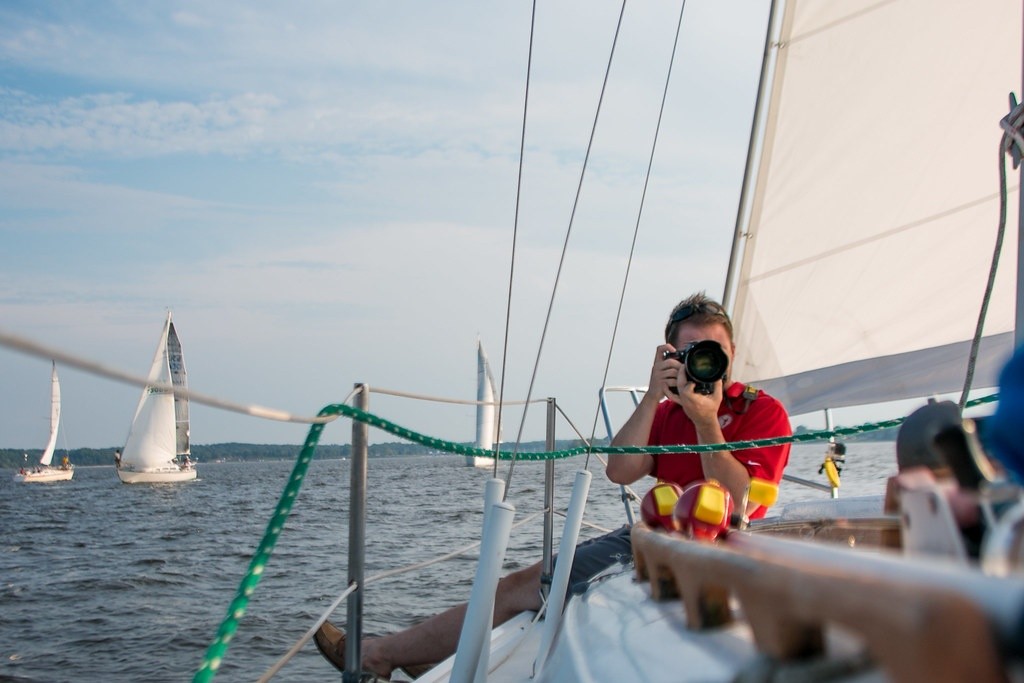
[313,619,391,683]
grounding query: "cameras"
[662,339,729,396]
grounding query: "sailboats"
[466,340,500,469]
[12,359,76,482]
[117,307,197,484]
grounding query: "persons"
[20,467,25,474]
[884,341,1024,534]
[114,449,121,467]
[62,455,69,468]
[312,287,793,683]
